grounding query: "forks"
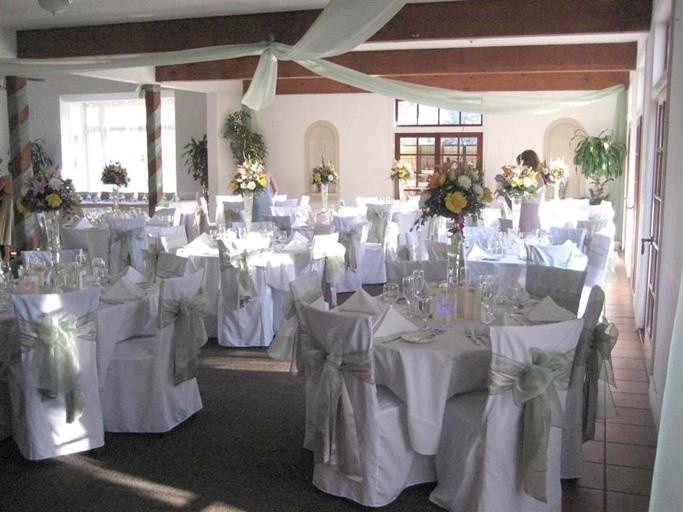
[463,326,480,345]
[474,327,489,347]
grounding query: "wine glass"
[380,266,528,332]
[201,220,289,254]
[0,248,110,313]
[464,229,550,259]
[289,202,344,225]
[374,191,417,211]
[73,189,148,224]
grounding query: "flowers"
[311,155,340,193]
[14,143,84,230]
[408,156,493,235]
[387,159,410,183]
[100,159,130,190]
[230,146,269,195]
[494,157,538,198]
[539,160,561,189]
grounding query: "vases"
[319,182,329,212]
[397,178,406,200]
[241,188,254,235]
[111,185,119,214]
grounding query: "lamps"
[37,0,72,16]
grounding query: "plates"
[523,306,575,326]
[401,328,436,345]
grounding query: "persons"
[516,149,545,201]
